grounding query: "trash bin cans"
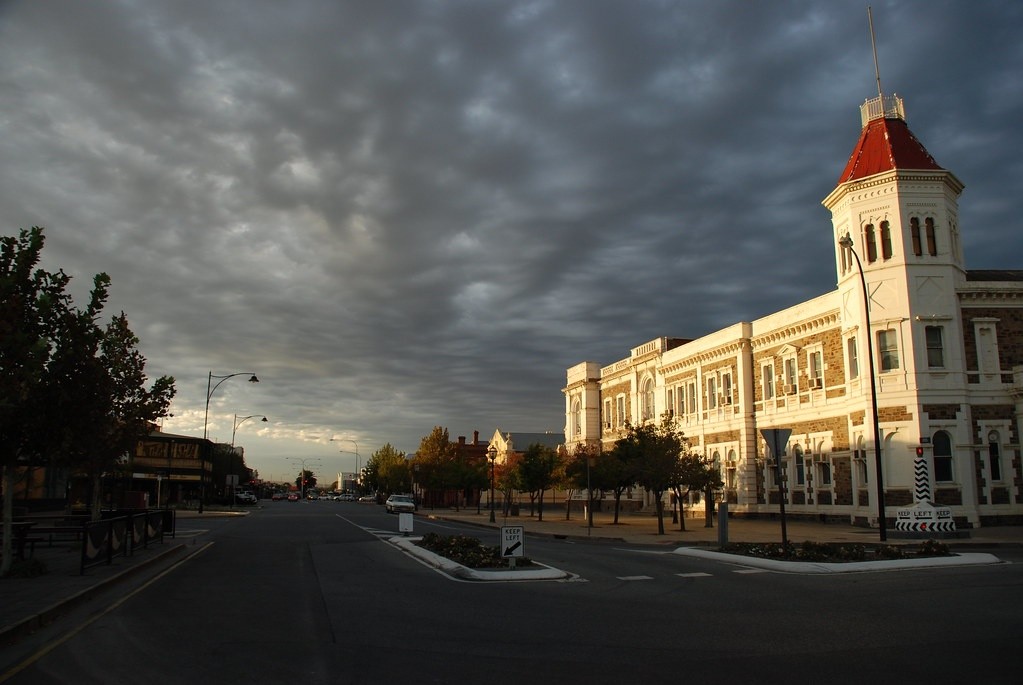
[511,502,519,517]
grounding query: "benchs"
[29,528,85,547]
[0,537,44,561]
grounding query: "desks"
[0,522,38,562]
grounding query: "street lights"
[330,439,358,502]
[841,238,887,543]
[489,446,497,523]
[339,451,361,499]
[155,472,166,508]
[230,414,268,509]
[286,457,322,503]
[199,372,260,514]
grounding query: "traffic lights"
[250,479,254,484]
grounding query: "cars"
[386,495,415,514]
[272,493,287,501]
[359,495,376,502]
[288,493,298,502]
[317,494,334,501]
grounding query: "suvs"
[237,491,258,504]
[333,494,355,502]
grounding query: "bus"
[307,488,318,499]
